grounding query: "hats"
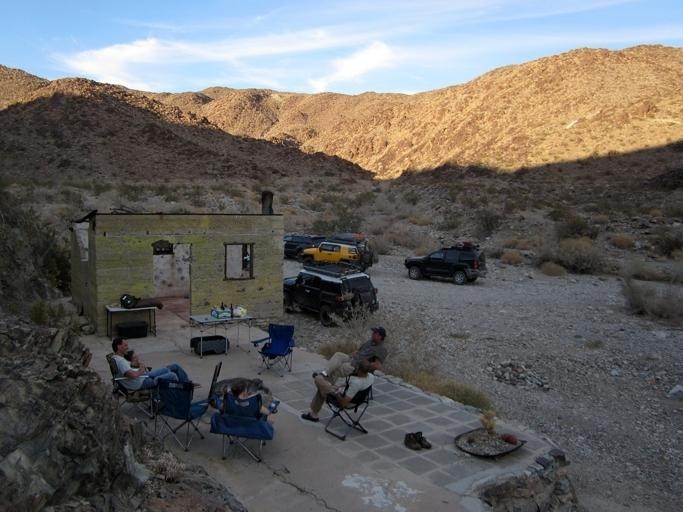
[350,359,370,371]
[370,326,386,337]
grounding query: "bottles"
[221,301,224,310]
[229,303,233,318]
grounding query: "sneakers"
[312,371,328,378]
[301,412,319,422]
[404,431,430,450]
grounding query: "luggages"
[115,321,148,339]
[189,335,229,355]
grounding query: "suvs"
[283,231,378,273]
[283,264,379,328]
[404,241,488,285]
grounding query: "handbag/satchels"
[119,293,137,308]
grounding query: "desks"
[104,304,159,341]
[188,313,257,359]
[210,377,270,410]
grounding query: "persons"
[108,336,178,392]
[301,357,375,423]
[123,350,201,388]
[217,381,272,418]
[311,326,388,386]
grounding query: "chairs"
[325,383,374,441]
[342,353,387,403]
[154,377,209,452]
[251,322,296,378]
[105,352,156,420]
[209,391,280,463]
